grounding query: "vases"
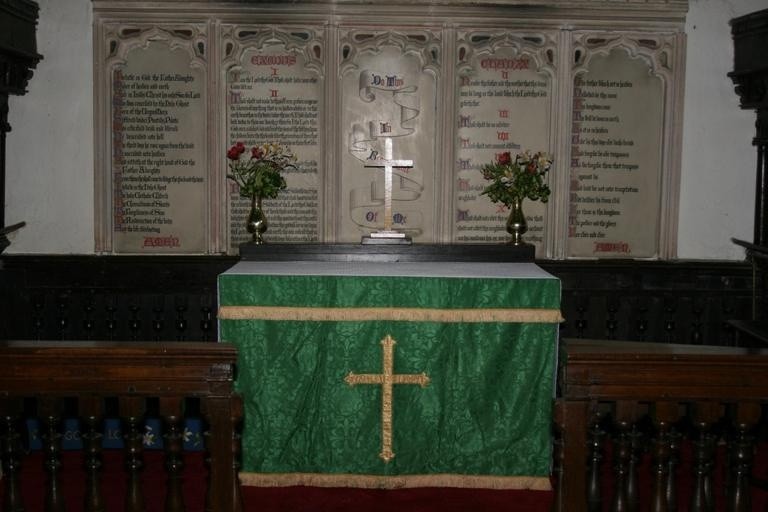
[505,197,529,246]
[247,194,268,245]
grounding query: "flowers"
[226,140,298,200]
[478,148,556,208]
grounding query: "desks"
[215,256,563,511]
